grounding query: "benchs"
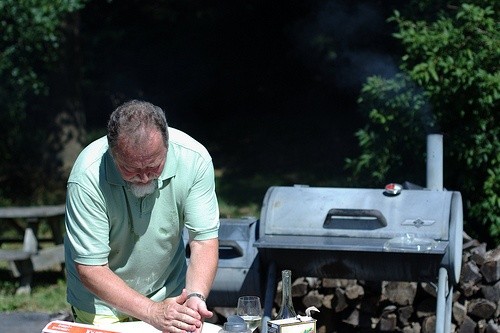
[0,248,39,260]
[1,236,63,244]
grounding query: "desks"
[0,202,65,295]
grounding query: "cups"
[237,296,262,330]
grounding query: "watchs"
[187,293,206,302]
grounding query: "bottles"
[275,270,298,319]
[220,316,252,333]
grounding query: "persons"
[64,100,221,333]
